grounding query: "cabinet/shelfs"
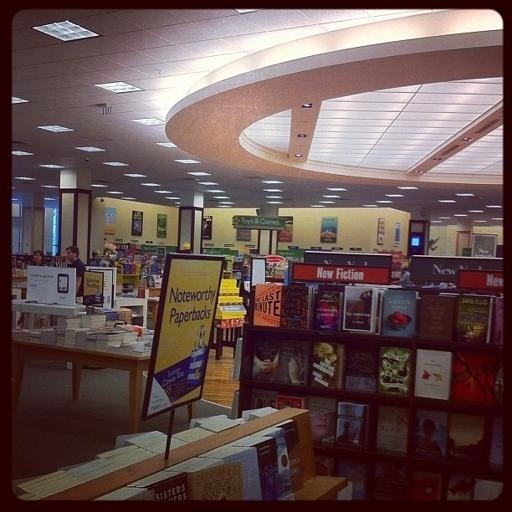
[11,405,347,501]
[237,278,509,502]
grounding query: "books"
[12,243,502,508]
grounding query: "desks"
[11,322,199,432]
